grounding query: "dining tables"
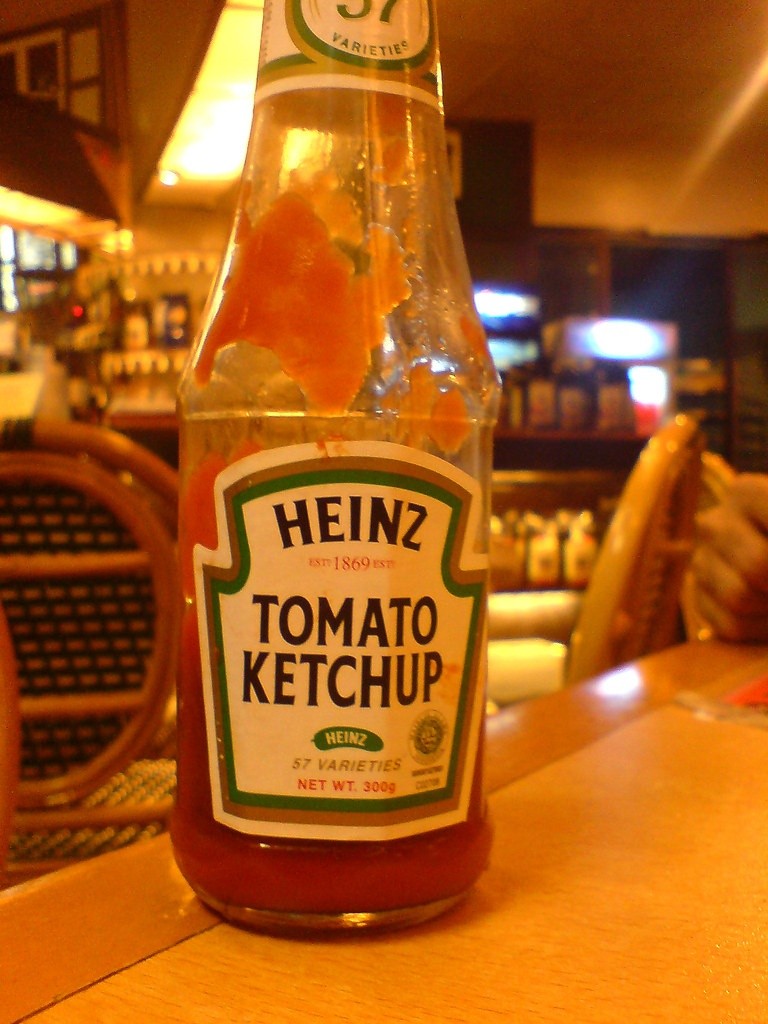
[0,634,768,1024]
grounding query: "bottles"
[508,358,634,433]
[492,508,599,592]
[169,0,502,930]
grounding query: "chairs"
[2,402,704,894]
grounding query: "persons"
[0,599,19,889]
[692,473,768,643]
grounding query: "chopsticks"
[699,451,737,508]
